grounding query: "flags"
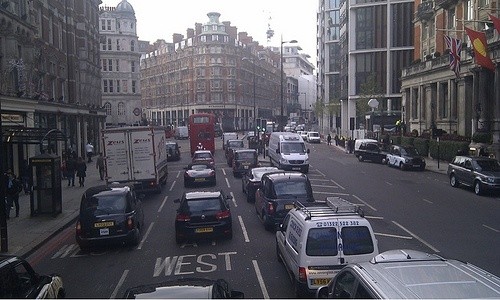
[464,27,495,71]
[486,12,500,37]
[444,35,462,79]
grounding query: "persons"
[334,135,338,146]
[5,168,17,208]
[6,172,20,218]
[328,134,331,145]
[96,152,106,180]
[86,141,94,163]
[66,155,77,187]
[20,159,30,194]
[77,157,86,187]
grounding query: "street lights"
[279,39,298,131]
[241,57,267,143]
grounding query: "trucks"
[103,127,168,194]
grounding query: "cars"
[165,140,182,161]
[0,254,65,300]
[162,126,188,140]
[447,155,500,196]
[218,119,323,232]
[75,184,144,247]
[354,141,391,164]
[386,145,425,171]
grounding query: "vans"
[315,250,500,299]
[355,140,377,151]
[274,202,379,300]
[268,132,311,171]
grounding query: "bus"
[188,114,215,156]
